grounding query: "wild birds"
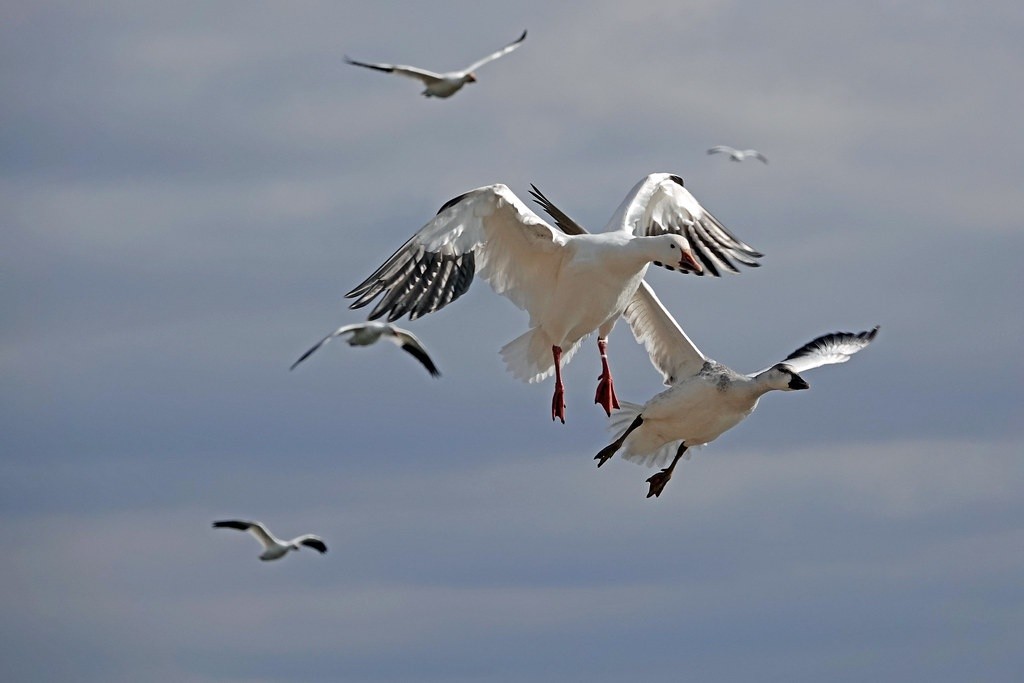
[706,146,768,166]
[212,520,327,561]
[343,30,528,99]
[290,322,443,380]
[343,173,766,424]
[529,183,880,499]
